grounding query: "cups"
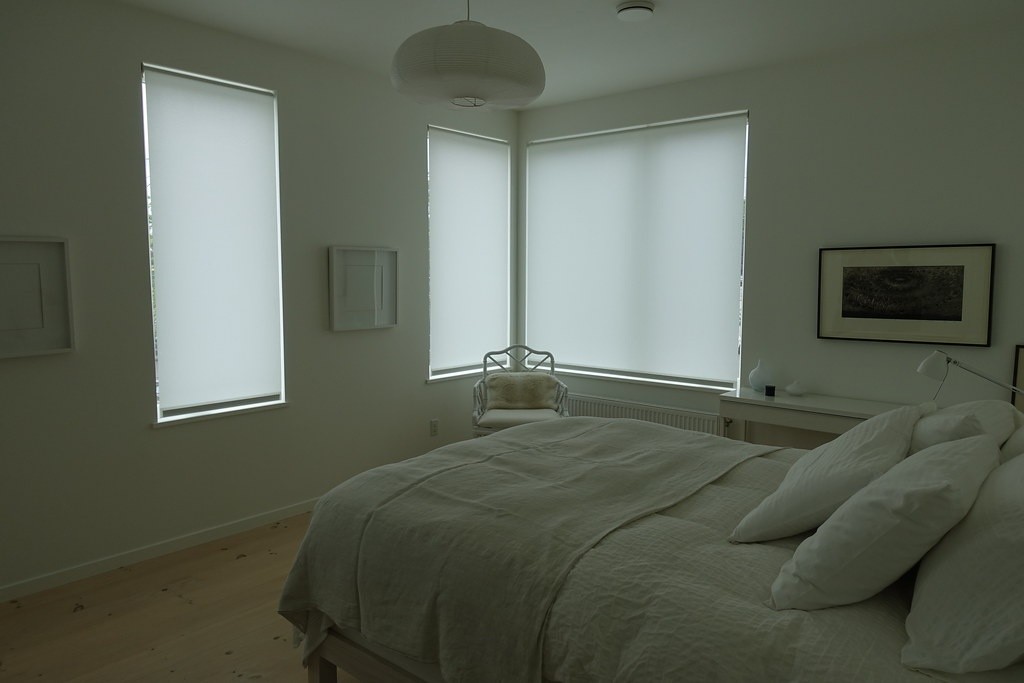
[766,385,775,396]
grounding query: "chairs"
[471,344,570,438]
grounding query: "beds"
[277,417,1024,683]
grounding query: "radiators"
[567,393,732,438]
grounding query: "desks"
[719,388,904,442]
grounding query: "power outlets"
[430,419,438,437]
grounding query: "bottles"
[784,380,807,396]
[748,360,769,391]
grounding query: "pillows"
[728,400,1024,674]
[477,409,560,428]
[485,371,560,411]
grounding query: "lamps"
[916,350,1024,395]
[390,0,546,110]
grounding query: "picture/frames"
[817,243,996,347]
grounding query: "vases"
[748,359,771,392]
